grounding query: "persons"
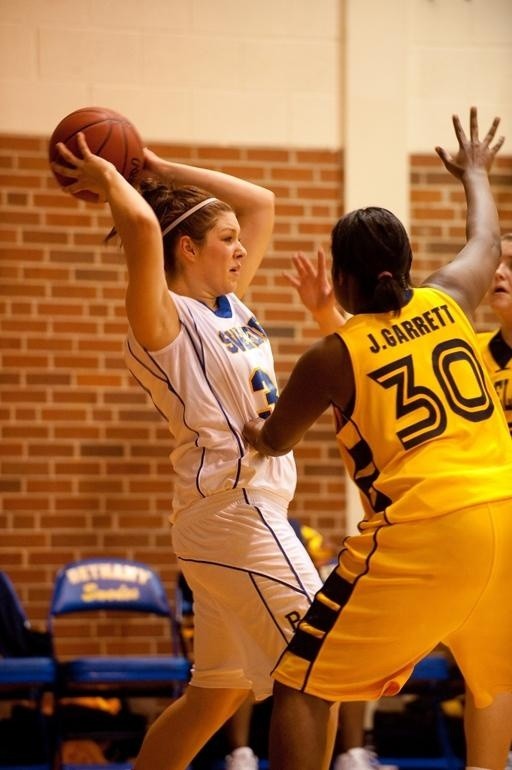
[176,515,400,770]
[234,101,512,770]
[47,129,326,770]
[278,232,512,441]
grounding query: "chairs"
[1,568,60,770]
[50,557,193,769]
[175,564,278,769]
[323,558,467,769]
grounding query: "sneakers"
[225,746,259,770]
[334,746,375,769]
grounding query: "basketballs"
[47,107,145,203]
